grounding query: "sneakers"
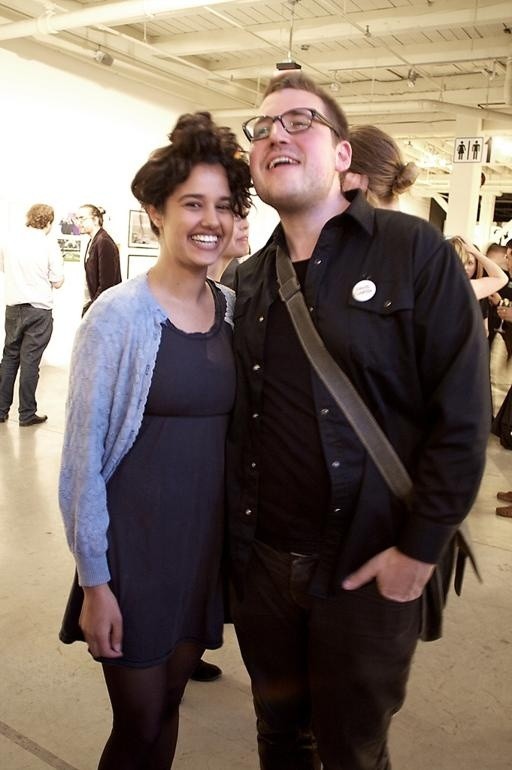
[492,418,512,450]
[192,657,223,682]
[0,412,48,426]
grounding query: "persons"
[200,201,251,285]
[441,233,511,520]
[220,70,498,770]
[53,109,260,770]
[75,204,122,317]
[335,123,422,217]
[0,202,67,428]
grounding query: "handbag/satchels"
[421,528,484,642]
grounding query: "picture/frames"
[127,209,160,249]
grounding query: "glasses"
[242,106,344,142]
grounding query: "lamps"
[406,67,418,88]
[276,1,302,71]
[481,58,497,81]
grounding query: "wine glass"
[493,296,511,334]
